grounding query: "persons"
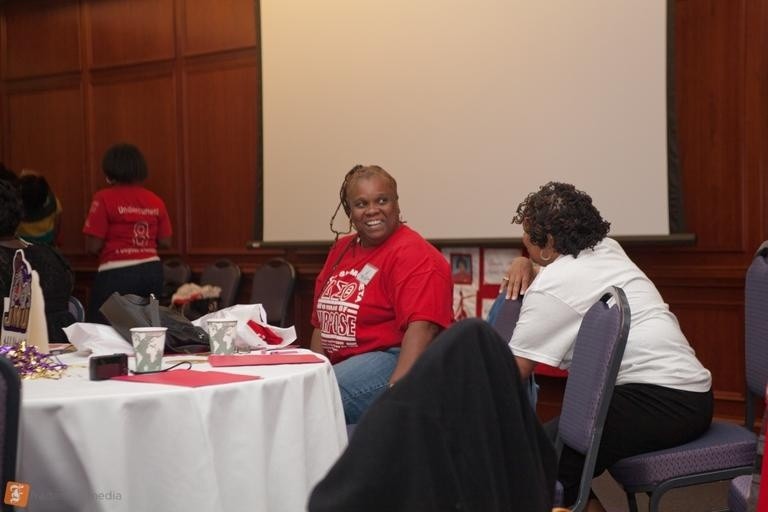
[498,181,714,512]
[452,260,472,284]
[0,164,77,344]
[82,142,174,325]
[309,164,454,427]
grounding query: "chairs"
[1,234,768,511]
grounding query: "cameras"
[89,353,129,379]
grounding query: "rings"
[503,276,509,281]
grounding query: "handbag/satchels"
[99,290,210,354]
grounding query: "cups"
[129,325,169,373]
[205,320,238,354]
